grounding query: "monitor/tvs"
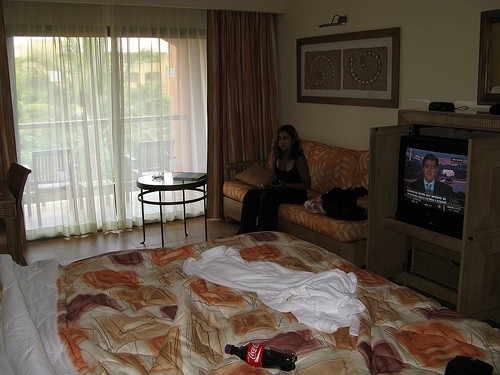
[394,134,469,240]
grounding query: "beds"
[0,232,500,375]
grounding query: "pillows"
[234,164,271,187]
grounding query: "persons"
[405,153,454,198]
[236,124,312,234]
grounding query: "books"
[173,172,206,181]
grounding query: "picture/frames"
[477,9,500,107]
[296,27,400,108]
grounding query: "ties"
[426,183,431,190]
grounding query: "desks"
[136,172,208,248]
[0,179,20,263]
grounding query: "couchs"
[221,140,370,268]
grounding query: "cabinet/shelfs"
[369,109,500,321]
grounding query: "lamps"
[319,14,347,29]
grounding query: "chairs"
[0,164,30,266]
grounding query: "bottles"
[225,341,299,372]
